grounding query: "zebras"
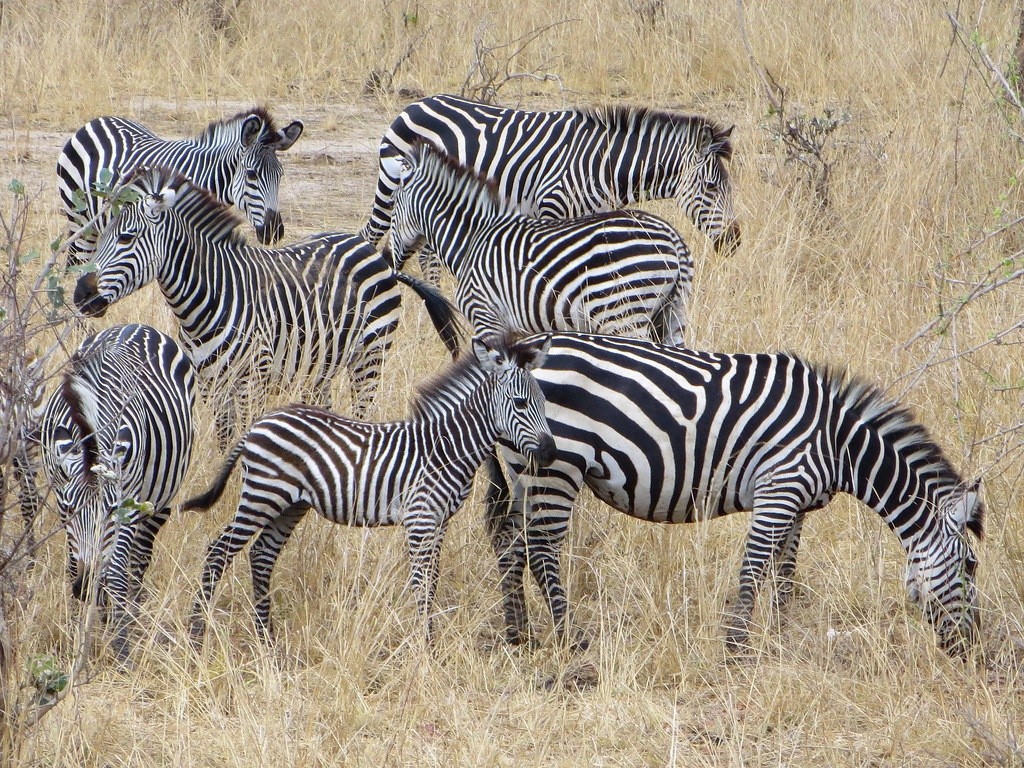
[0,92,988,690]
